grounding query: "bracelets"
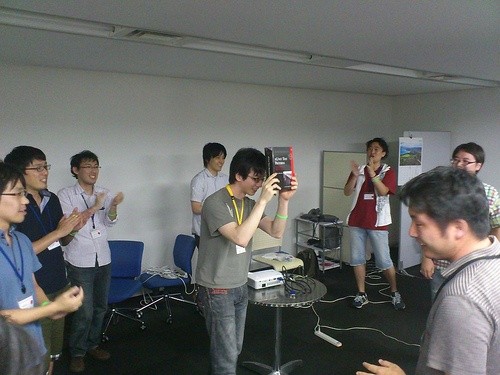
[69,230,79,236]
[275,213,289,219]
[41,299,48,306]
[371,175,380,184]
[108,211,116,216]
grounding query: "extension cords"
[314,331,342,348]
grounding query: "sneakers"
[389,292,405,311]
[353,291,371,308]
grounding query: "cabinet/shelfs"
[295,218,343,274]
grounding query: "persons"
[354,167,500,375]
[190,142,230,250]
[344,137,405,311]
[0,162,83,375]
[57,151,123,374]
[195,148,299,375]
[419,142,500,304]
[3,145,80,375]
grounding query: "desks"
[242,274,327,375]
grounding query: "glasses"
[21,164,52,173]
[78,163,101,170]
[0,190,29,198]
[244,174,266,184]
[449,158,477,168]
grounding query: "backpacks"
[296,249,319,280]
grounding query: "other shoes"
[70,355,85,373]
[87,346,112,361]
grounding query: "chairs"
[100,240,144,344]
[136,234,199,318]
[251,228,304,276]
